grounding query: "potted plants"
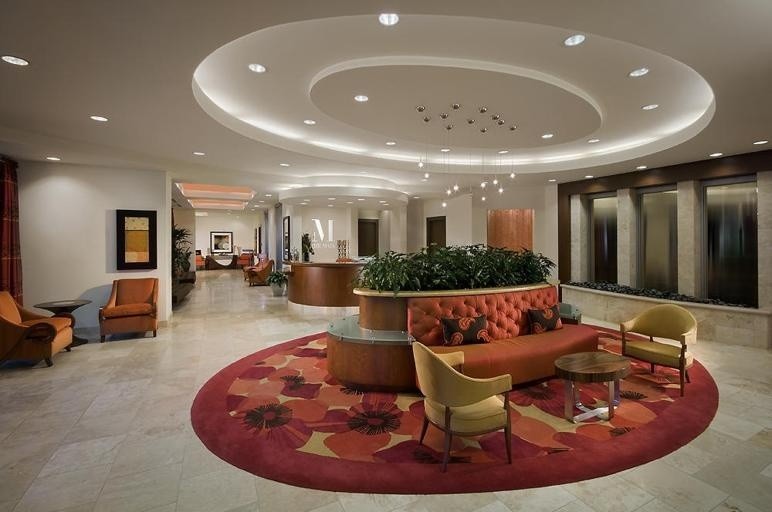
[349,241,562,333]
[266,269,290,297]
[169,205,194,292]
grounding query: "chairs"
[97,277,161,345]
[557,298,583,326]
[191,248,276,289]
[616,301,698,398]
[410,340,514,476]
[0,289,75,369]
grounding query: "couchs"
[401,282,600,396]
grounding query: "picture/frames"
[210,230,234,254]
[283,215,291,261]
[114,206,160,271]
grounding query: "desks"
[286,260,370,309]
[551,350,637,421]
[32,296,93,350]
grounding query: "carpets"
[192,311,723,496]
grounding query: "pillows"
[438,312,492,347]
[525,303,563,336]
